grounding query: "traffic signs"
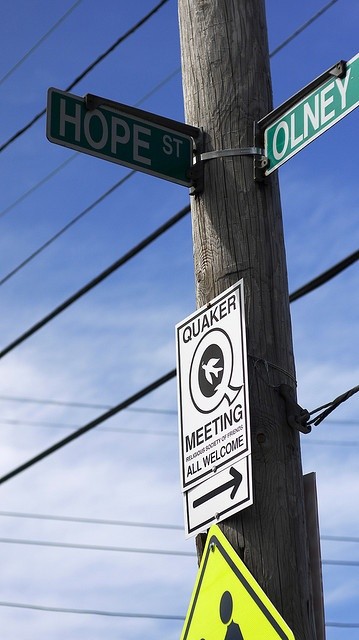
[174,278,266,539]
[45,86,204,194]
[251,54,357,182]
